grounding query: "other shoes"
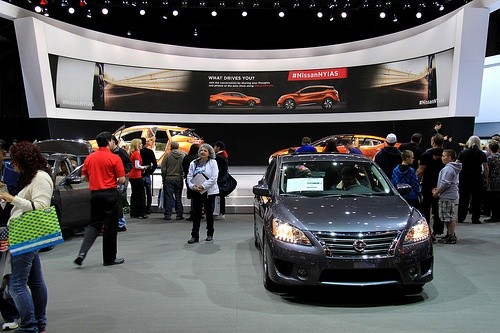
[458,218,496,224]
[137,210,205,221]
[218,214,225,219]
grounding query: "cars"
[269,134,401,164]
[210,92,261,107]
[252,153,434,295]
[87,125,204,168]
[33,153,92,251]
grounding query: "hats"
[386,133,397,143]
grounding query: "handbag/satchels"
[7,200,64,256]
[217,172,237,197]
[158,189,175,208]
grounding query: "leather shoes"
[188,238,198,243]
[74,257,83,265]
[205,236,212,241]
[103,258,124,266]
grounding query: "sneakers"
[438,234,457,244]
[2,318,21,329]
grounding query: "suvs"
[277,85,340,111]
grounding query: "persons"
[159,142,189,220]
[127,139,150,219]
[392,150,423,208]
[457,136,489,224]
[432,150,466,244]
[0,142,63,333]
[74,132,126,266]
[214,142,228,220]
[60,161,71,177]
[483,134,500,222]
[0,140,26,331]
[399,121,448,237]
[187,143,219,244]
[289,137,361,155]
[438,128,500,154]
[183,144,205,220]
[139,137,158,215]
[336,167,362,190]
[110,134,132,231]
[373,133,402,181]
